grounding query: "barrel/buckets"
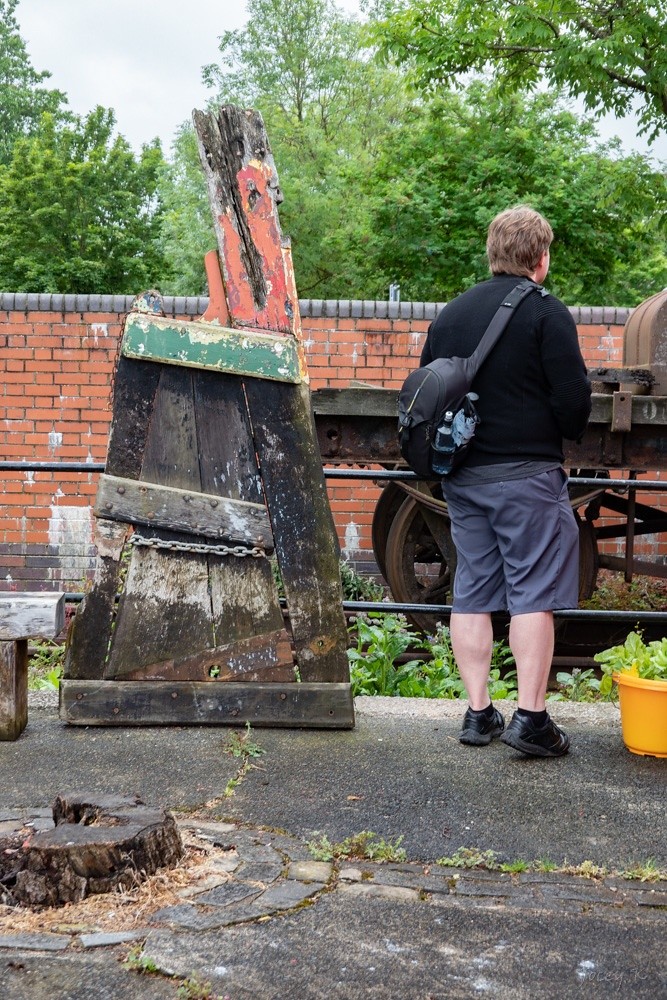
[612,671,667,759]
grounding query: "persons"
[419,208,593,753]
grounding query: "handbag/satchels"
[397,356,479,482]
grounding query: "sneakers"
[459,705,505,746]
[500,711,570,756]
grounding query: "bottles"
[432,410,457,475]
[452,392,479,452]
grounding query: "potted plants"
[595,622,667,759]
